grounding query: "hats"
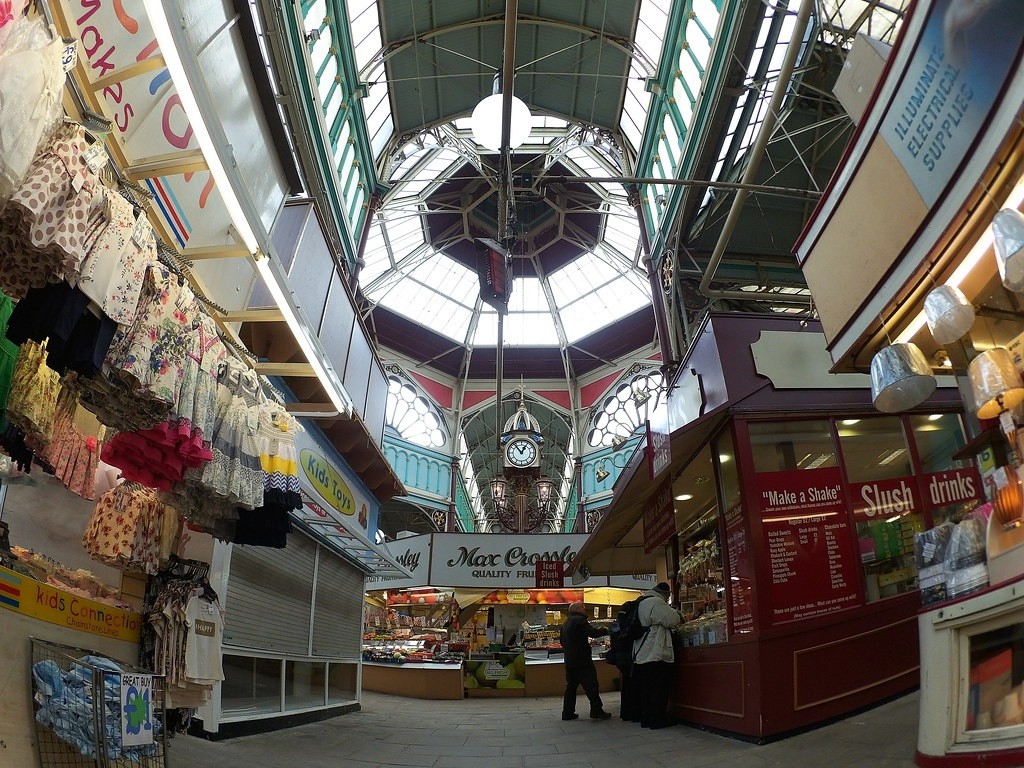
[654,582,670,593]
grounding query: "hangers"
[119,479,146,493]
[158,555,216,604]
[218,349,249,374]
[192,295,219,336]
[158,244,194,286]
[111,179,155,220]
[63,110,114,166]
[232,356,268,405]
[261,391,305,433]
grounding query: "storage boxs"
[832,33,893,128]
[900,513,922,523]
[903,538,913,547]
[396,531,419,540]
[905,545,914,553]
[902,529,923,540]
[901,521,924,531]
[881,523,905,558]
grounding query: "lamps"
[572,558,591,585]
[596,458,631,483]
[967,347,1024,468]
[633,375,680,408]
[922,260,975,345]
[471,70,532,153]
[979,178,1024,293]
[870,315,937,413]
[252,247,265,261]
[612,433,642,451]
[490,473,557,533]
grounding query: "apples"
[528,590,582,603]
[484,591,507,603]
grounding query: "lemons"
[464,654,524,688]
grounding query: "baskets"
[30,636,168,768]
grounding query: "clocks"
[505,437,539,468]
[517,609,525,618]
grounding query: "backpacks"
[605,618,634,666]
[617,594,654,640]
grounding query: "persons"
[608,582,682,729]
[559,600,612,721]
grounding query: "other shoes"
[590,707,612,718]
[562,712,579,720]
